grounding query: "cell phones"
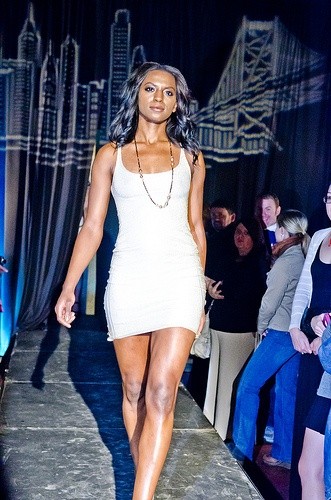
[322,314,331,327]
